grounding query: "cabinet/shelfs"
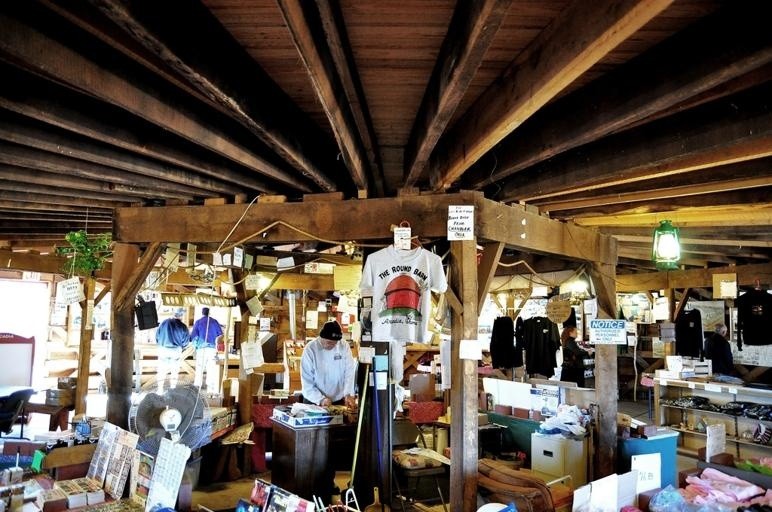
[653,378,772,474]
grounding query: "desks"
[21,393,68,432]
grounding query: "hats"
[319,320,343,341]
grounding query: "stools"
[401,466,448,512]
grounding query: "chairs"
[478,457,574,512]
[0,389,33,436]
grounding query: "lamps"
[651,220,681,271]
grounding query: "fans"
[128,377,211,457]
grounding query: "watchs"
[190,307,223,398]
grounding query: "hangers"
[738,278,772,300]
[367,221,441,260]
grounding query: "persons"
[703,322,735,376]
[156,305,190,395]
[301,320,360,410]
[560,326,597,389]
